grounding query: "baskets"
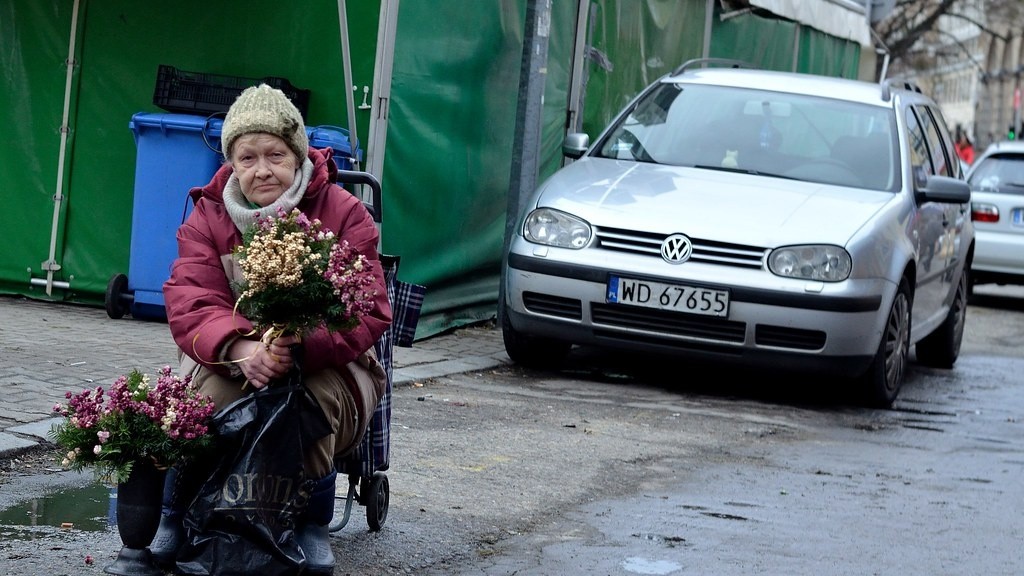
[152,64,309,125]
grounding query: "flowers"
[231,208,379,339]
[48,365,213,483]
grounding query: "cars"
[960,139,1024,307]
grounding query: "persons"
[955,128,975,163]
[140,84,394,575]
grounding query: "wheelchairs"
[100,168,400,576]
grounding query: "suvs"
[495,53,978,412]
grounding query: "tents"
[1,0,870,351]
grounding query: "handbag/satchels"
[172,377,335,576]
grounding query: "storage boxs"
[152,65,312,125]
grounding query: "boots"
[294,467,336,574]
[149,462,203,560]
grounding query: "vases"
[104,466,166,576]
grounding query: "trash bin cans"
[105,109,362,324]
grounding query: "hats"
[221,83,307,165]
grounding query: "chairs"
[697,113,785,168]
[828,133,885,188]
